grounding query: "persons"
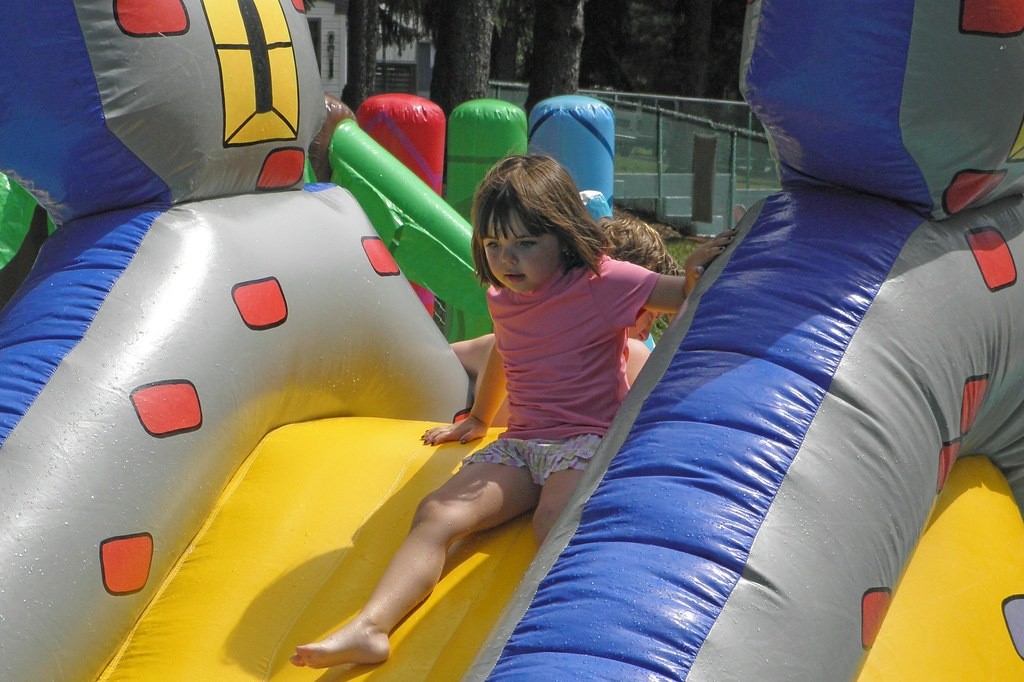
[451,216,686,428]
[289,156,741,669]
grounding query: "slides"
[0,185,1023,681]
